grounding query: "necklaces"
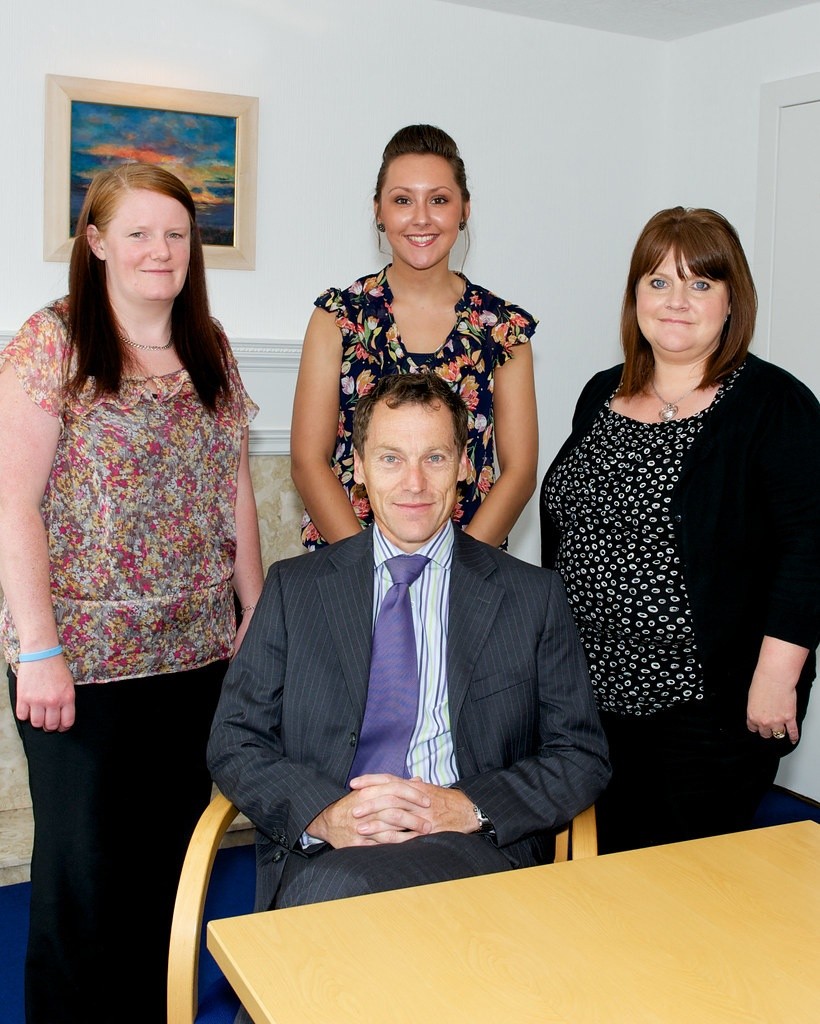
[650,376,694,422]
[116,325,174,349]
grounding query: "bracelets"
[240,604,256,615]
[13,644,65,661]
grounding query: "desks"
[205,818,819,1024]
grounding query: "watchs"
[473,803,493,833]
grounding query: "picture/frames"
[43,72,260,272]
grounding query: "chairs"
[165,794,598,1024]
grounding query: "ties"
[345,554,431,792]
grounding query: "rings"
[772,728,786,739]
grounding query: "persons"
[207,373,614,912]
[0,162,267,1021]
[539,207,819,857]
[289,124,539,554]
